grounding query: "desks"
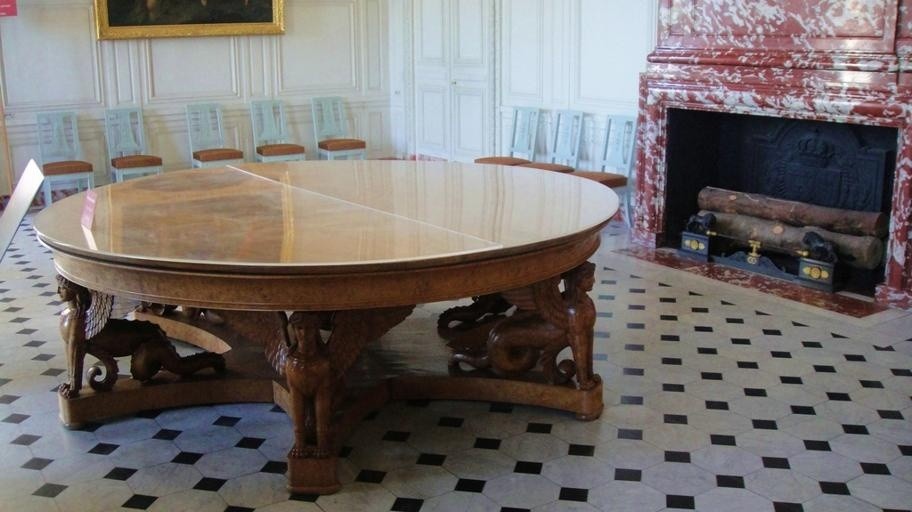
[33,159,622,494]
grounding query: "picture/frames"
[93,0,286,41]
